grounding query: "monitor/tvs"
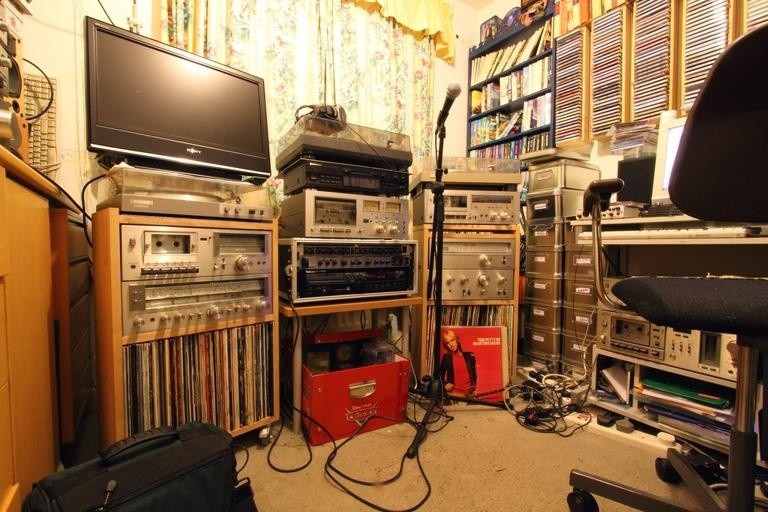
[83,16,271,184]
[651,116,688,205]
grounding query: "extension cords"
[518,368,571,406]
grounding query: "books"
[595,361,733,446]
[121,321,275,438]
[423,304,516,403]
[468,17,554,236]
[555,0,768,162]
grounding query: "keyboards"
[578,226,761,239]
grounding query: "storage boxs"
[523,159,600,398]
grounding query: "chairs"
[566,22,767,512]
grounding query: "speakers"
[0,23,30,165]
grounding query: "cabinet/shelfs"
[91,206,281,457]
[1,142,82,512]
[464,1,554,173]
[408,224,521,386]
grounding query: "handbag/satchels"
[22,421,237,511]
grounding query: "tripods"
[407,128,514,457]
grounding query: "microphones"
[435,82,461,135]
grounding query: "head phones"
[295,105,346,136]
